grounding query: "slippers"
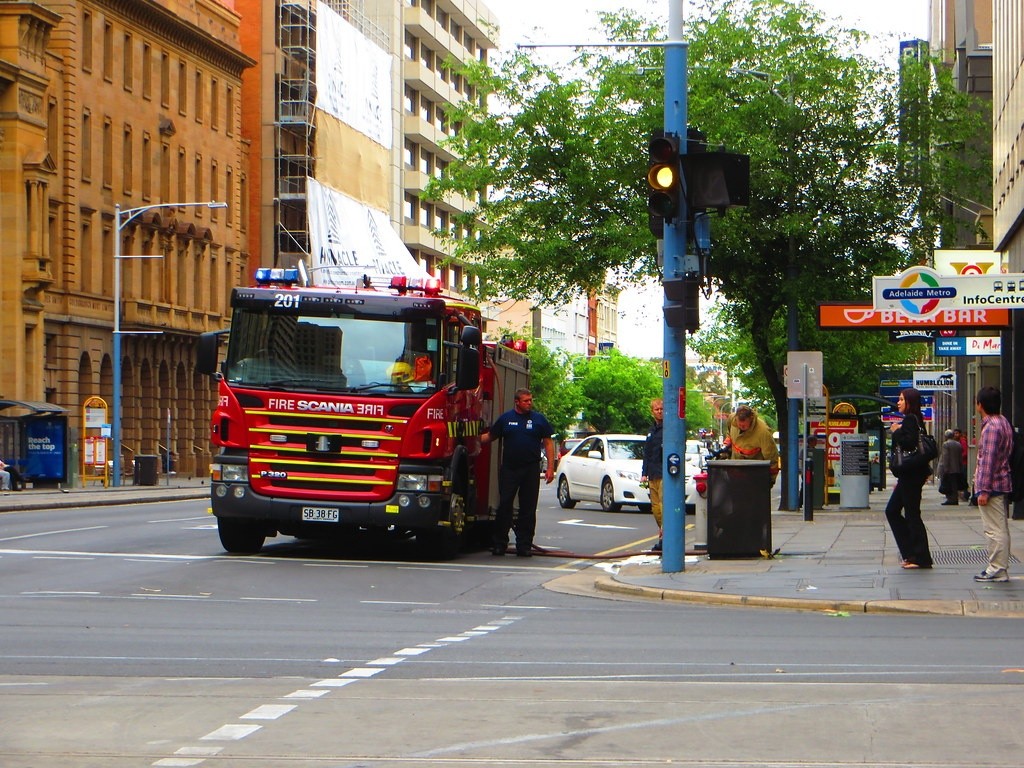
[900,560,932,569]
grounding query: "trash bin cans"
[706,458,770,560]
[135,454,156,486]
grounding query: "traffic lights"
[648,136,680,218]
[662,270,700,334]
[683,150,751,206]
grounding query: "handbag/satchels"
[889,414,921,478]
[914,415,939,461]
[938,473,952,494]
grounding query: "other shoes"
[941,499,958,505]
[651,539,661,551]
[516,548,532,557]
[492,543,505,555]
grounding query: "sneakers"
[974,568,1009,582]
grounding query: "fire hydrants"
[693,471,708,550]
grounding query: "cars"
[685,440,709,471]
[555,434,701,514]
[558,439,582,459]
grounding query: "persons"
[641,398,663,551]
[937,429,967,505]
[162,452,176,474]
[799,437,817,507]
[0,460,10,491]
[885,388,935,569]
[973,386,1013,581]
[721,406,779,490]
[482,388,555,557]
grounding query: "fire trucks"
[195,268,531,560]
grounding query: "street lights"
[638,66,800,512]
[112,200,229,487]
[571,356,611,383]
